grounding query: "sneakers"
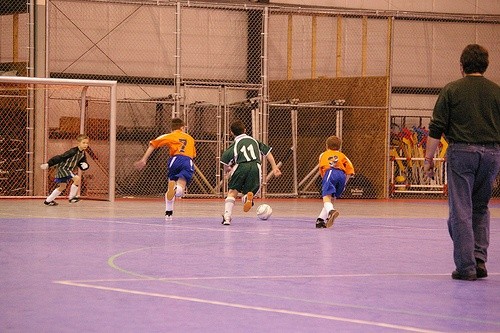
[165,210,172,220]
[167,180,177,200]
[222,213,231,225]
[326,210,339,227]
[452,267,487,281]
[69,198,79,203]
[244,192,254,212]
[316,218,326,228]
[44,200,58,206]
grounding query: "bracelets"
[425,157,434,159]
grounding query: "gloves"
[40,164,48,170]
[80,162,88,169]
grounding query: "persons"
[315,136,355,228]
[40,134,90,206]
[422,43,500,281]
[219,122,281,225]
[135,117,197,221]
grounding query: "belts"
[449,140,500,146]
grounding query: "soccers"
[255,203,273,220]
